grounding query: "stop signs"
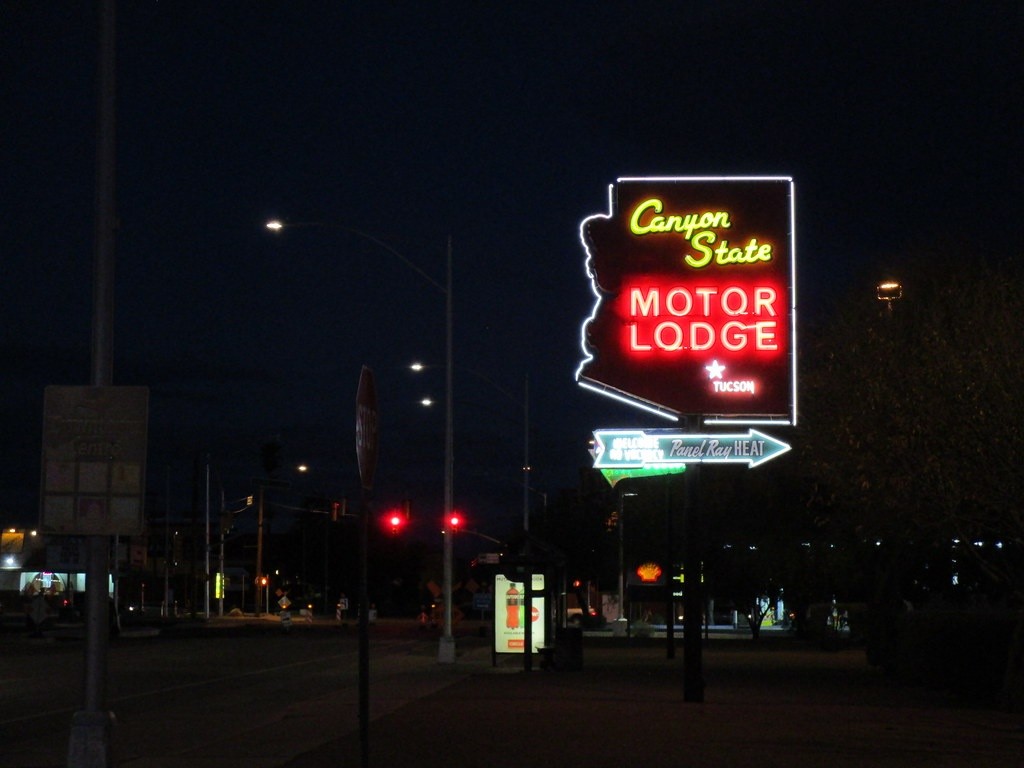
[353,366,384,494]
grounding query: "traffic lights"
[381,507,409,536]
[445,510,467,536]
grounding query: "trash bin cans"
[557,628,587,671]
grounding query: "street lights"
[266,215,461,676]
